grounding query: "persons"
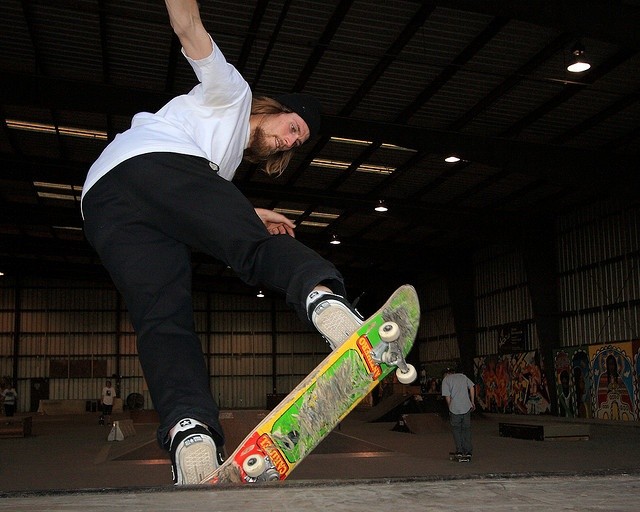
[80,1,369,485]
[98,381,116,429]
[2,384,19,417]
[441,367,476,458]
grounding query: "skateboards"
[449,452,472,462]
[199,285,421,485]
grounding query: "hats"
[276,92,322,144]
[441,367,455,374]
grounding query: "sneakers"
[466,452,471,456]
[455,451,462,456]
[168,417,224,487]
[305,288,362,350]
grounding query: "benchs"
[1,415,32,437]
